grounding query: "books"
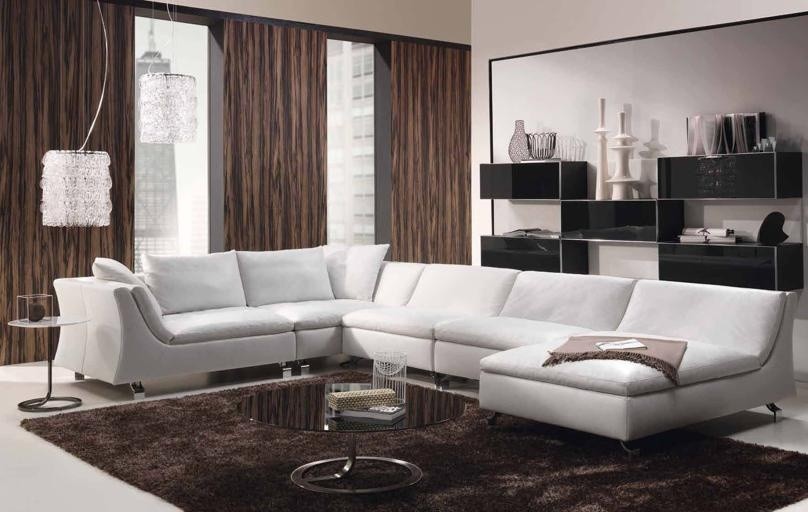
[677,233,737,245]
[502,228,560,239]
[343,405,407,421]
[344,415,405,426]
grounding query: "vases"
[508,120,530,163]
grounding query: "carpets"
[20,369,808,512]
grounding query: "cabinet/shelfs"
[479,151,803,292]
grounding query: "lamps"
[39,0,112,229]
[137,0,198,146]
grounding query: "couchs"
[51,259,799,482]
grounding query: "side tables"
[8,316,96,413]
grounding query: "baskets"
[525,133,557,159]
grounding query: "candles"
[600,97,606,128]
[650,118,660,143]
[618,111,625,134]
[623,103,632,136]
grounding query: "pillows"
[324,238,392,303]
[92,256,164,320]
[140,249,246,315]
[235,246,336,307]
